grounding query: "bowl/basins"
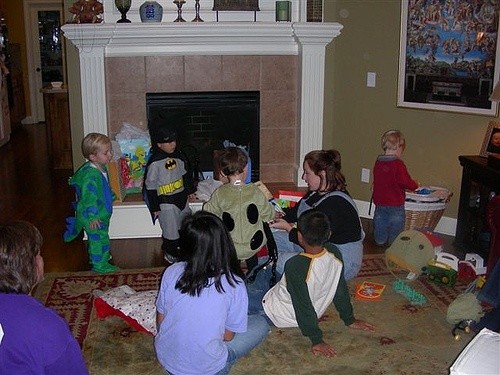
[51,81,63,88]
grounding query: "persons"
[488,131,500,154]
[64,133,120,272]
[0,220,90,375]
[202,146,276,276]
[373,130,419,246]
[268,149,366,285]
[153,211,269,375]
[142,131,197,264]
[245,210,376,359]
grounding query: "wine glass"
[173,0,187,22]
[114,0,132,23]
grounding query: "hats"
[152,127,178,144]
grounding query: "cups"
[276,1,290,23]
[307,0,323,22]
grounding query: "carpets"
[34,251,498,375]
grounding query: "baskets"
[404,201,447,232]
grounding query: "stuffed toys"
[384,229,442,279]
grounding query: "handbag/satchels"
[446,293,484,340]
[383,229,434,283]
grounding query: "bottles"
[140,1,163,23]
[461,318,476,334]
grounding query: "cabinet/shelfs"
[40,82,71,170]
[454,155,500,279]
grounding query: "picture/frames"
[480,120,500,158]
[397,0,500,116]
